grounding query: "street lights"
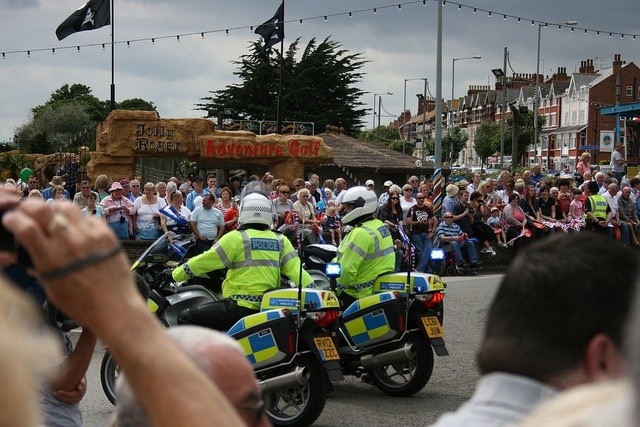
[534,20,578,171]
[373,91,393,143]
[402,77,428,156]
[449,55,481,176]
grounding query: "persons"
[229,177,242,196]
[43,176,70,200]
[109,324,268,427]
[568,189,583,222]
[435,211,483,269]
[184,176,209,211]
[159,191,191,253]
[24,176,41,197]
[561,185,572,220]
[422,233,640,427]
[378,191,418,273]
[216,187,234,235]
[94,174,109,200]
[522,185,545,240]
[133,183,163,240]
[485,207,509,249]
[164,194,316,331]
[578,171,593,195]
[399,185,418,236]
[332,177,347,195]
[293,188,321,227]
[323,186,343,245]
[73,181,100,209]
[512,171,518,185]
[601,183,630,248]
[262,172,271,187]
[289,178,313,204]
[424,179,434,192]
[119,179,135,201]
[321,179,336,204]
[155,182,169,208]
[325,187,397,307]
[312,175,318,181]
[549,187,566,219]
[418,184,434,208]
[271,185,294,226]
[304,180,312,191]
[310,178,320,202]
[502,194,536,256]
[479,179,503,219]
[577,153,592,179]
[453,191,476,239]
[468,190,499,255]
[378,180,394,207]
[270,179,286,199]
[467,172,478,198]
[6,179,23,198]
[531,163,546,182]
[190,192,225,251]
[522,169,536,187]
[43,318,92,427]
[129,179,143,201]
[610,142,628,185]
[441,185,458,216]
[100,181,135,240]
[582,183,614,237]
[166,182,178,205]
[81,192,107,222]
[250,174,259,181]
[536,186,556,234]
[365,180,375,192]
[628,178,640,205]
[406,192,439,274]
[225,197,239,231]
[617,186,640,246]
[598,179,611,195]
[219,184,228,189]
[170,178,180,188]
[591,168,598,177]
[408,176,419,198]
[455,180,467,198]
[513,177,525,196]
[203,174,221,199]
[179,172,195,195]
[594,173,604,189]
[564,165,568,174]
[46,185,66,201]
[0,181,246,427]
[495,180,519,209]
[498,170,510,189]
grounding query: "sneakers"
[488,247,496,256]
[480,248,491,255]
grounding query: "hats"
[365,179,374,185]
[49,175,66,186]
[446,184,458,197]
[491,207,499,212]
[192,176,204,181]
[120,179,130,186]
[109,181,124,191]
[384,180,393,186]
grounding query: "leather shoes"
[471,261,482,268]
[462,262,468,267]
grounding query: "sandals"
[505,246,508,248]
[498,241,506,247]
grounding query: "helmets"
[239,193,273,228]
[240,180,270,200]
[339,185,377,223]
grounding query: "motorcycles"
[100,229,327,427]
[300,243,450,397]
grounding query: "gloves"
[159,268,172,281]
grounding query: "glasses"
[486,186,490,187]
[282,191,290,193]
[392,197,398,199]
[444,215,454,218]
[82,187,90,189]
[405,188,413,191]
[29,180,35,183]
[303,195,309,197]
[132,184,139,187]
[417,196,424,199]
[410,179,418,182]
[459,187,466,190]
[90,197,96,199]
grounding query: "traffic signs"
[600,130,614,153]
